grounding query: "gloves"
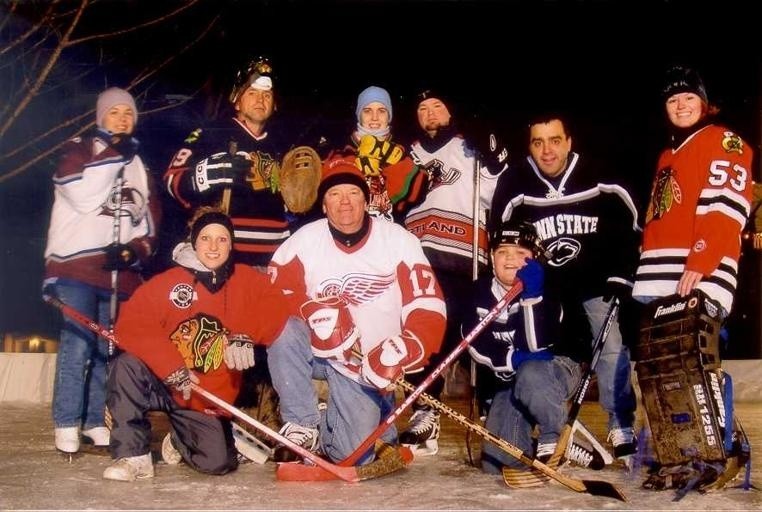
[465,126,511,175]
[107,185,148,226]
[183,152,254,197]
[353,135,407,178]
[516,259,544,299]
[512,348,555,370]
[165,364,200,401]
[407,404,442,457]
[102,242,138,271]
[223,333,256,371]
[274,422,320,463]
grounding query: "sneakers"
[606,427,639,461]
[80,425,111,447]
[102,449,155,481]
[643,429,741,490]
[161,432,184,466]
[534,435,605,474]
[54,426,81,453]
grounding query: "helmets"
[229,54,278,105]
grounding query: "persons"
[627,69,757,489]
[321,86,433,232]
[452,221,593,473]
[489,110,641,458]
[103,208,292,482]
[260,144,451,465]
[162,59,332,449]
[392,89,517,450]
[40,86,161,454]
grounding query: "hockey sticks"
[503,295,628,493]
[276,281,524,482]
[104,172,123,430]
[349,351,629,502]
[464,153,482,465]
[56,301,414,482]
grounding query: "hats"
[318,153,370,206]
[661,63,709,103]
[492,217,544,259]
[356,86,393,124]
[412,87,454,117]
[95,87,138,128]
